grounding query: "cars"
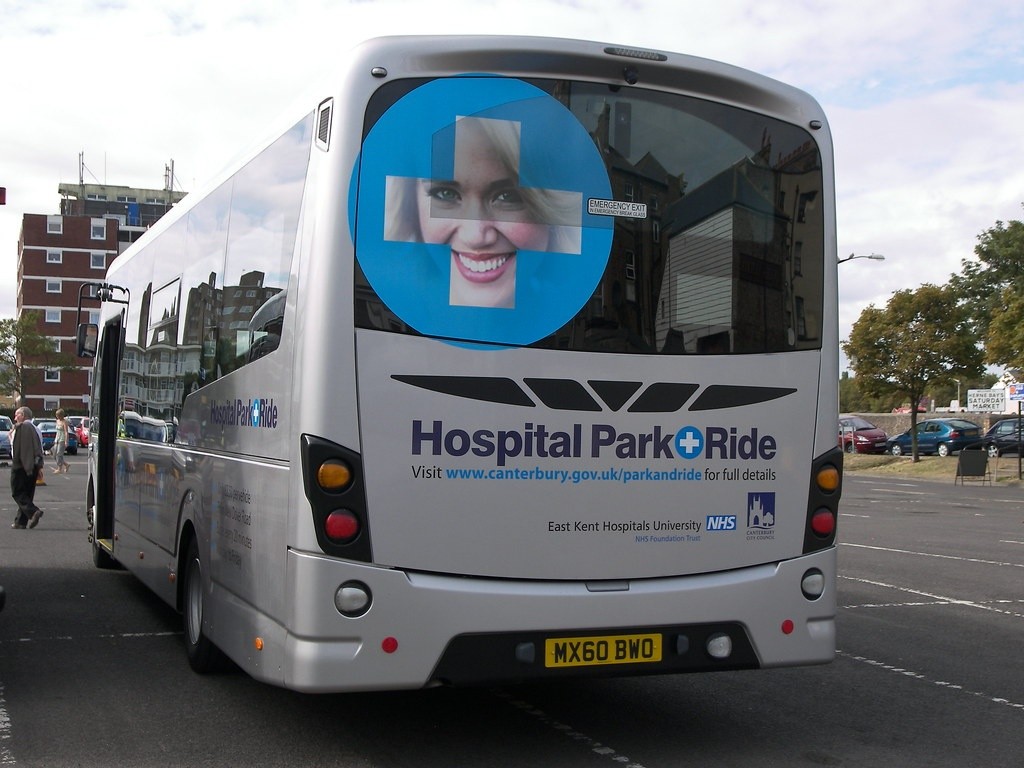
[37,421,78,455]
[76,417,90,448]
[32,417,58,426]
[885,418,986,457]
[65,414,88,432]
[838,414,889,456]
[891,403,928,414]
[981,417,1024,458]
[0,415,17,462]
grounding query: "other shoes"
[65,463,70,472]
[11,523,26,529]
[28,509,43,528]
[54,470,63,473]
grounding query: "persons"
[8,407,44,529]
[383,116,583,309]
[52,409,71,474]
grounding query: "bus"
[76,29,849,696]
